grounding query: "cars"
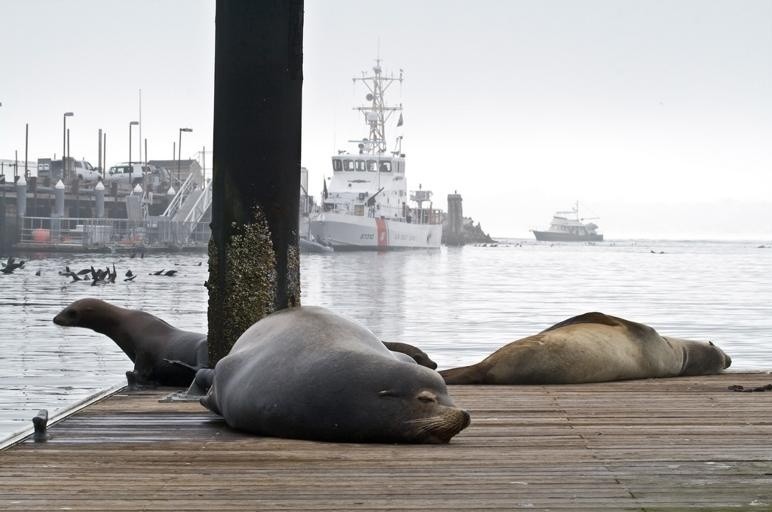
[47,160,150,191]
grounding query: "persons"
[401,201,411,218]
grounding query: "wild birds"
[149,269,165,277]
[35,270,41,276]
[58,264,139,287]
[0,254,28,275]
[166,270,178,277]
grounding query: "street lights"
[63,112,73,157]
[128,121,139,181]
[178,127,192,181]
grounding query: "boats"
[532,199,603,241]
[311,35,442,249]
